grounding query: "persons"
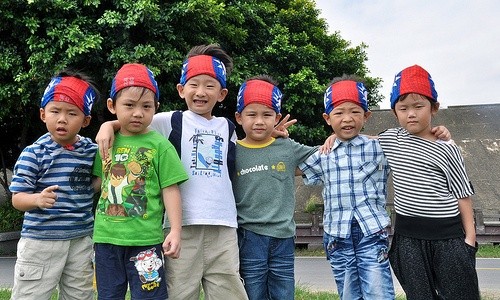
[93,62,189,300]
[96,44,297,300]
[319,64,479,300]
[232,74,322,300]
[9,68,99,300]
[295,74,453,300]
